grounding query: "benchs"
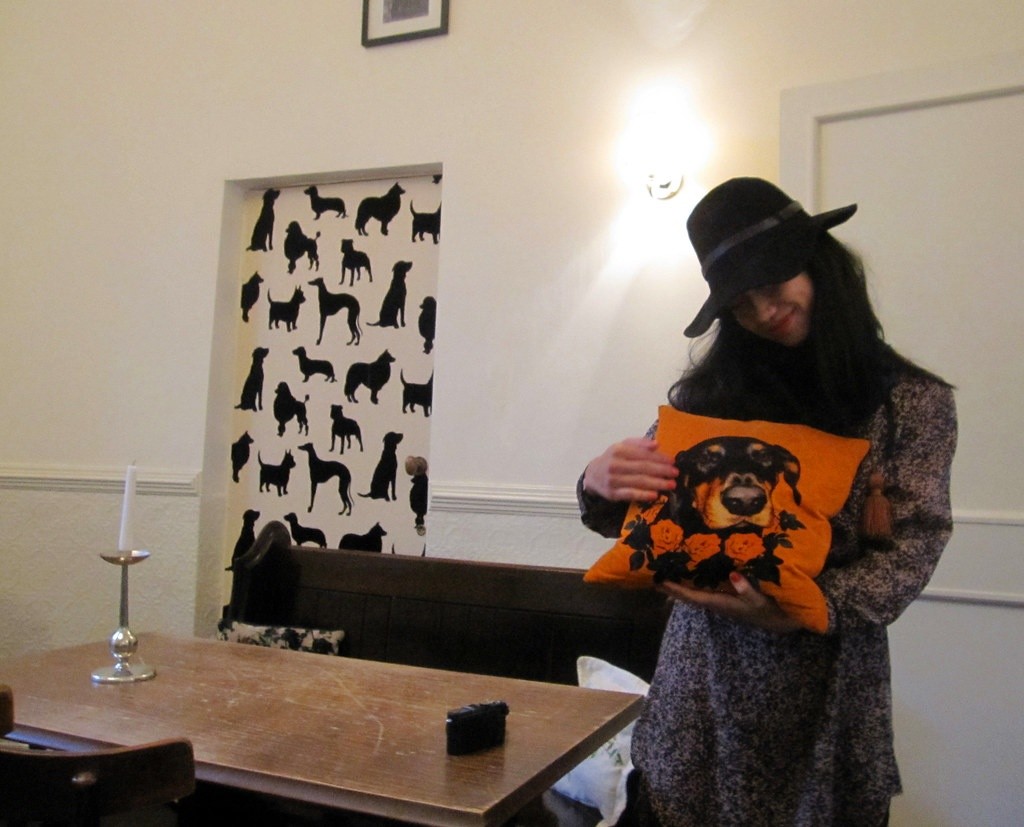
[222,550,672,827]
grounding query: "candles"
[119,459,139,551]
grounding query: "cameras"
[445,700,510,757]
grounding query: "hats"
[683,176,859,339]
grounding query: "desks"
[0,638,650,827]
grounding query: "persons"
[578,177,960,827]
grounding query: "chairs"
[0,736,197,827]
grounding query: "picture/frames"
[361,0,449,48]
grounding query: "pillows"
[582,404,872,637]
[214,615,347,657]
[553,654,650,810]
